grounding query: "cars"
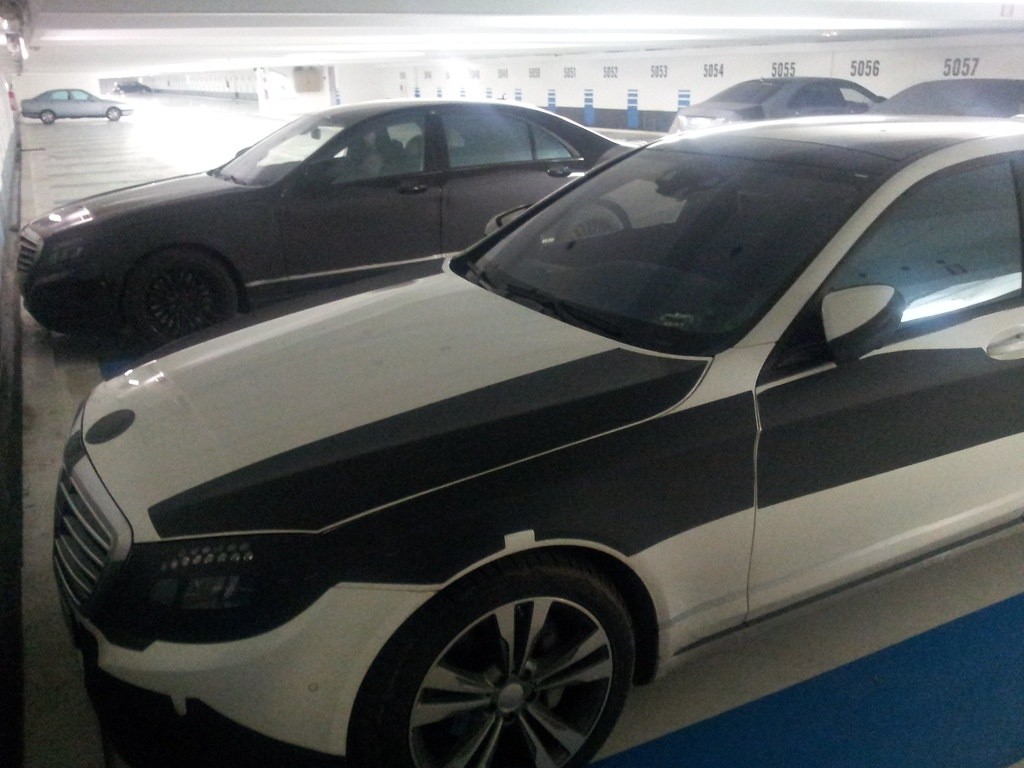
[17,96,640,354]
[672,76,889,135]
[868,78,1024,115]
[53,112,1024,768]
[20,88,133,125]
[112,80,152,95]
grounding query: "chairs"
[395,135,422,170]
[356,131,391,172]
